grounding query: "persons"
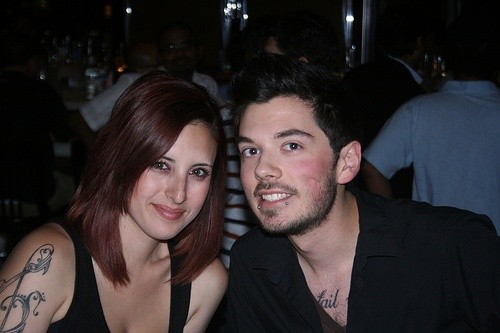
[361,10,500,237]
[282,11,335,67]
[342,4,428,198]
[0,31,75,215]
[74,20,218,151]
[0,70,229,333]
[218,15,286,271]
[208,54,500,333]
